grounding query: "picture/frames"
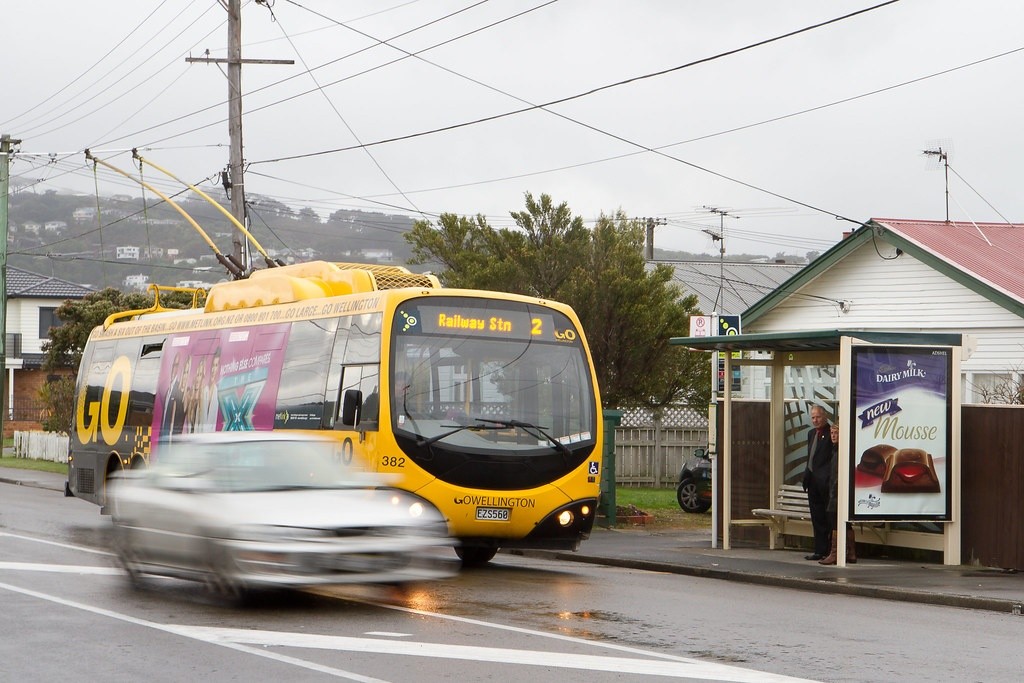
[848,346,953,524]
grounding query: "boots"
[818,530,837,565]
[845,529,857,563]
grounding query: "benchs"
[751,483,891,545]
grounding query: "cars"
[677,441,712,515]
[104,427,464,608]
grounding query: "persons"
[802,406,857,565]
[159,346,221,436]
[395,371,413,415]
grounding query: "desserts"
[855,444,940,493]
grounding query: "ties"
[808,432,819,472]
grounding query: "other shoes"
[804,553,830,560]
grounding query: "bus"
[63,149,606,567]
